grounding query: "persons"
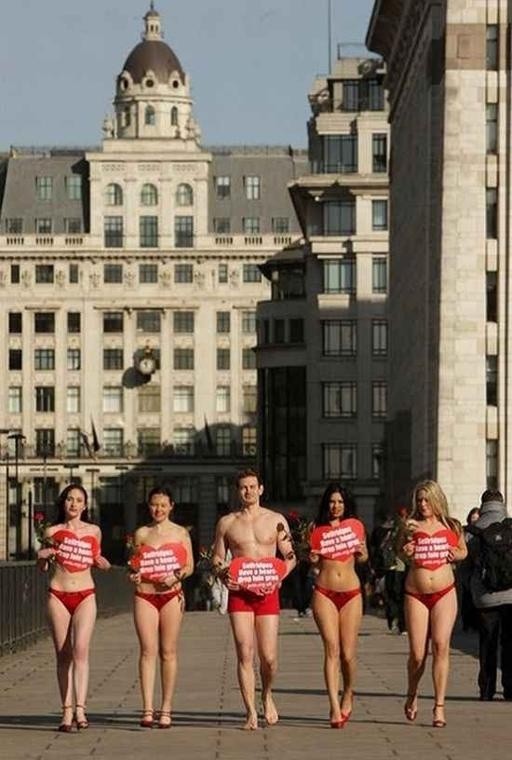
[457,507,480,636]
[212,469,297,730]
[305,483,369,729]
[465,489,511,703]
[210,542,232,615]
[286,515,313,619]
[36,484,112,733]
[126,488,194,730]
[351,500,409,637]
[394,481,468,727]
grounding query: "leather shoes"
[59,704,88,732]
[140,710,172,728]
[405,693,417,721]
[328,708,353,729]
[431,703,445,728]
